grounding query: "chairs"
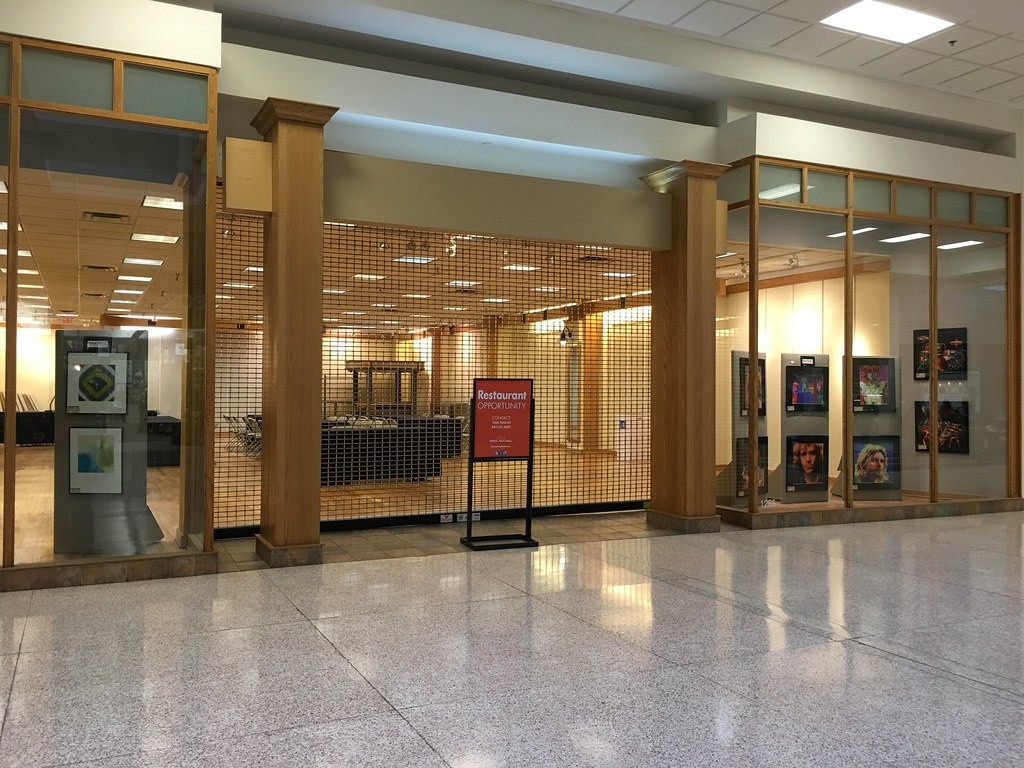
[327,413,398,431]
[221,414,262,463]
[421,413,470,454]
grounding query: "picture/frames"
[65,351,130,416]
[739,357,766,417]
[914,401,970,455]
[853,435,900,490]
[853,358,896,412]
[913,327,968,381]
[68,426,123,496]
[737,436,768,497]
[785,435,828,492]
[786,366,829,411]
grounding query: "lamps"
[558,326,572,346]
